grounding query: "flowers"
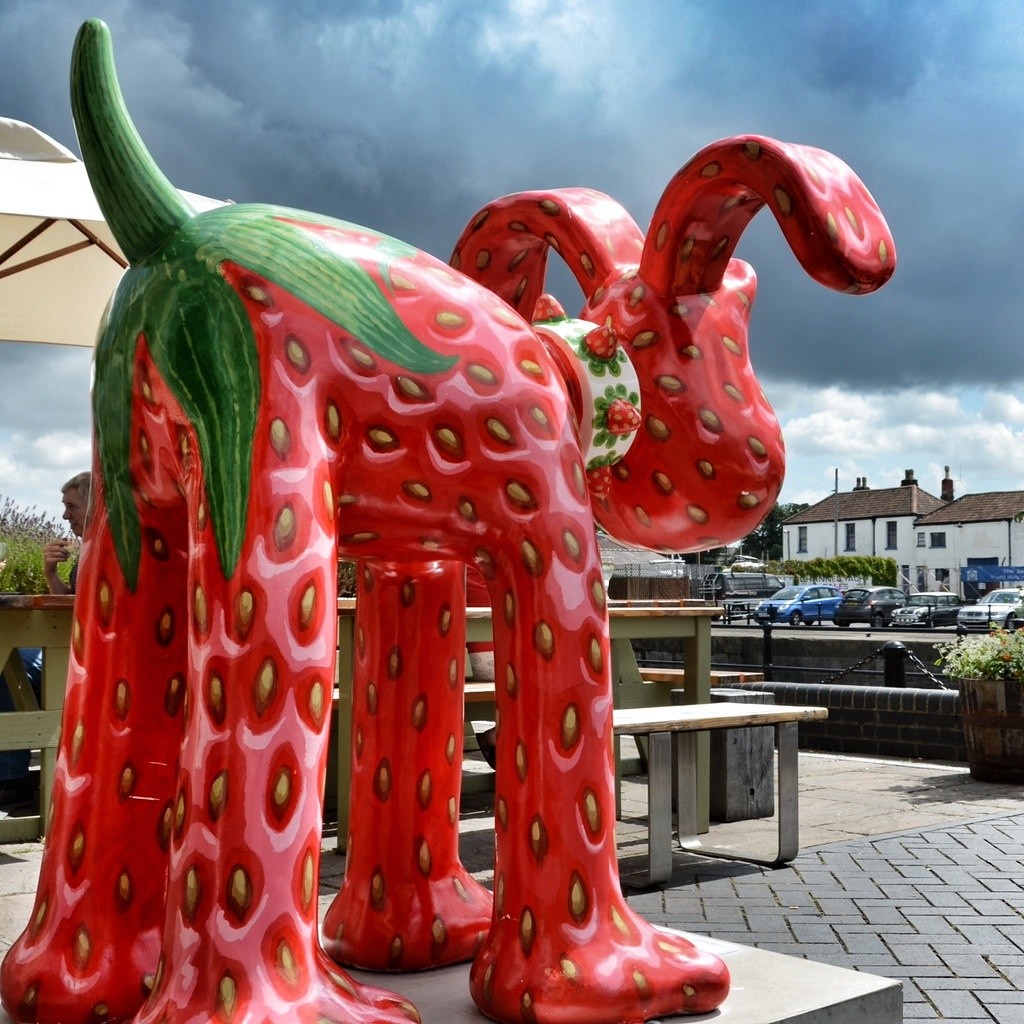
[930,621,1024,683]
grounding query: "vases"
[955,675,1024,785]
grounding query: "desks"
[0,592,728,858]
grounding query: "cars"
[957,588,1024,630]
[835,586,920,629]
[891,592,967,631]
[754,585,844,627]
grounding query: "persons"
[43,472,91,595]
[0,647,42,795]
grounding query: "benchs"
[635,665,766,687]
[610,702,829,888]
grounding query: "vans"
[731,555,765,568]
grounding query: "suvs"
[701,571,800,621]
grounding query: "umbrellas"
[0,116,231,349]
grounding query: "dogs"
[1,16,901,1024]
